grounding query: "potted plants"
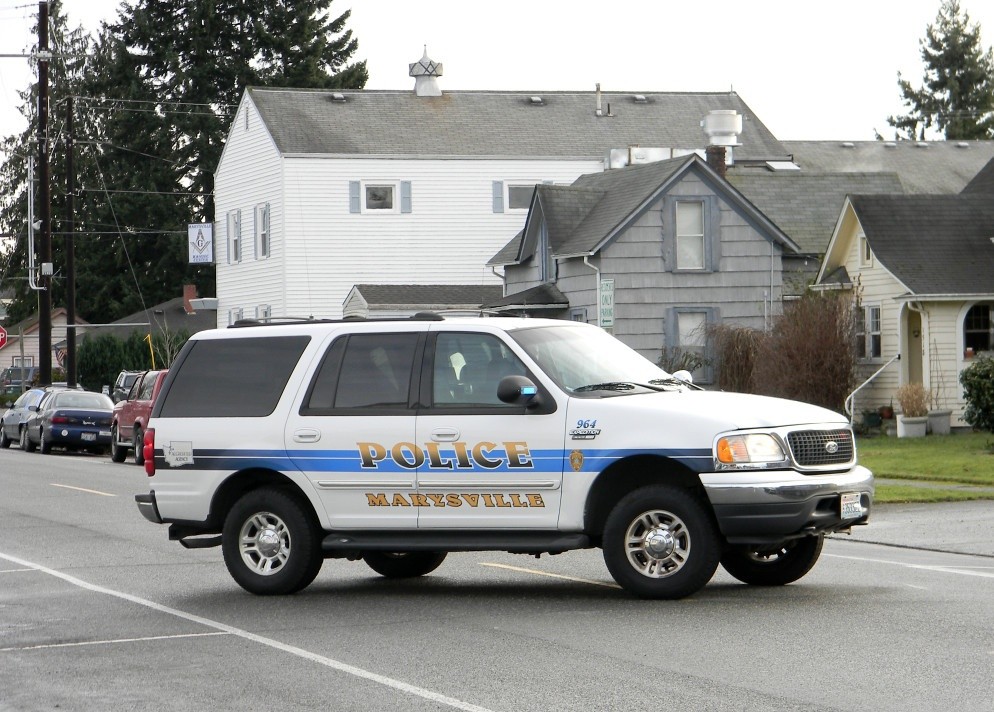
[896,381,929,437]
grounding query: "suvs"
[134,308,874,598]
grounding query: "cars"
[0,369,170,465]
